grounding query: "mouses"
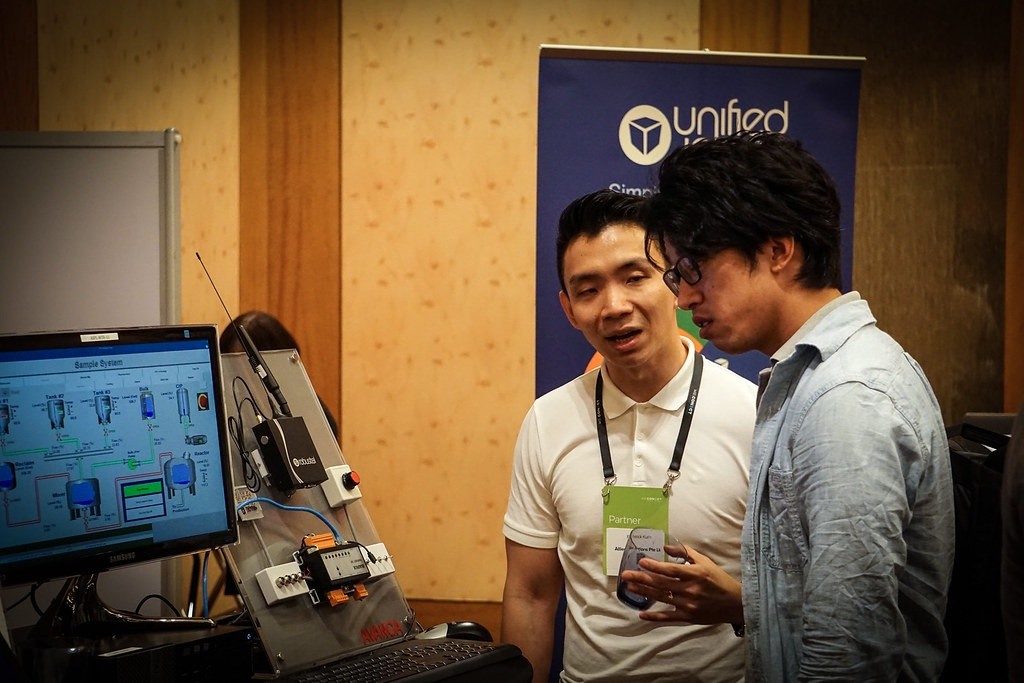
[413,622,493,642]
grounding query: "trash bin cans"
[942,422,1011,615]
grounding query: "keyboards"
[275,636,524,683]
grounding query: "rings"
[666,591,674,604]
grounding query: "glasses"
[662,244,731,296]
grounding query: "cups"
[616,527,688,610]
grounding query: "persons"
[638,130,955,683]
[500,190,759,683]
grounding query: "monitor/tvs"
[0,323,242,648]
